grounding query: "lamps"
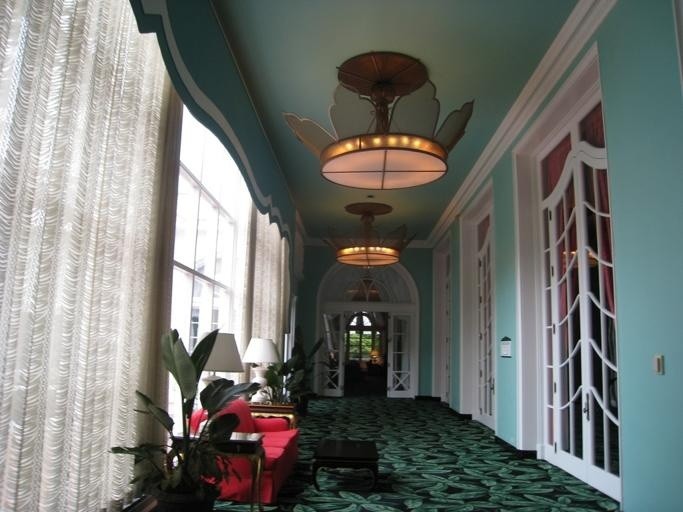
[243,337,278,402]
[336,202,401,266]
[319,51,448,189]
[192,332,244,387]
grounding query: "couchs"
[170,399,299,503]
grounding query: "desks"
[248,402,298,429]
[167,433,265,511]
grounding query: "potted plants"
[108,327,261,512]
